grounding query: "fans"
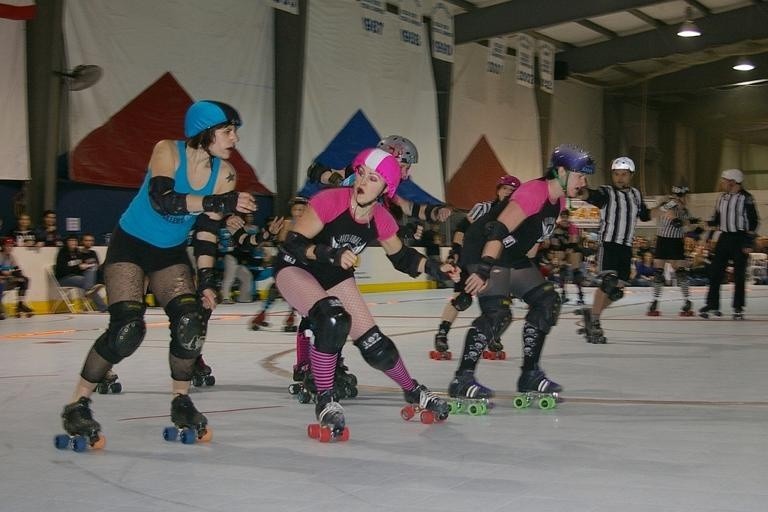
[48,65,104,91]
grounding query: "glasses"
[356,169,380,187]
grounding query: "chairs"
[46,264,94,314]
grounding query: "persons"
[0,210,110,317]
[244,135,596,442]
[53,99,257,451]
[184,195,310,333]
[575,156,760,344]
[365,208,767,308]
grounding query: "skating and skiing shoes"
[590,323,607,344]
[647,300,746,320]
[14,303,32,316]
[54,355,216,452]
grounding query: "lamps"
[732,56,759,71]
[677,7,702,38]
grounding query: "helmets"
[352,149,401,198]
[378,135,418,164]
[185,100,242,138]
[498,145,744,197]
